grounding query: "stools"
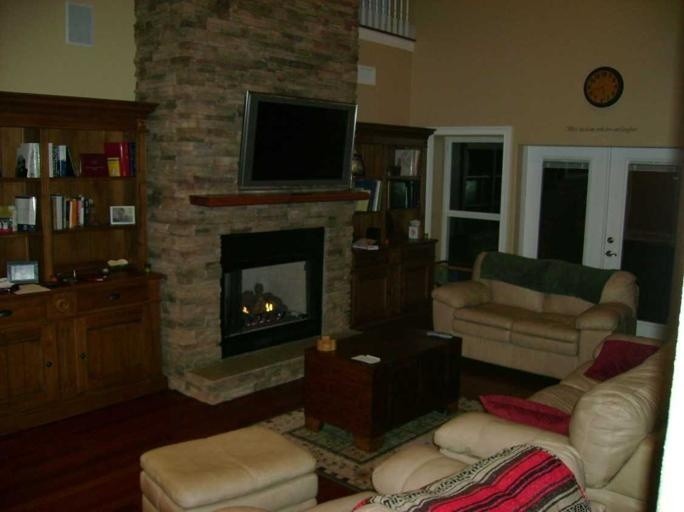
[137,423,317,512]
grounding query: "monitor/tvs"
[238,90,358,186]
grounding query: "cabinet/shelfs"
[353,121,435,325]
[0,91,167,435]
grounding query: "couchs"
[429,249,640,380]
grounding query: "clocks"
[582,67,624,108]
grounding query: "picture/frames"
[105,201,136,227]
[65,2,95,49]
[5,260,38,283]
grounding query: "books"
[353,146,422,214]
[11,140,136,231]
[352,236,380,250]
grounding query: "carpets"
[252,395,485,494]
